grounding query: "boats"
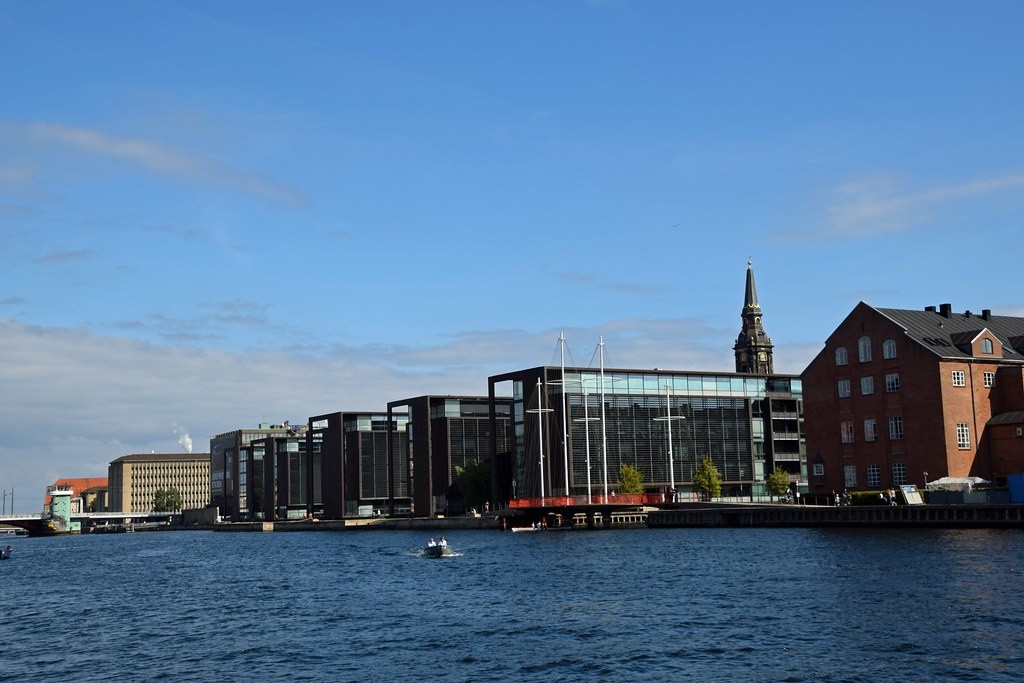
[0,527,29,538]
[0,546,12,560]
[424,545,457,557]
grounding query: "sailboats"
[513,324,649,528]
